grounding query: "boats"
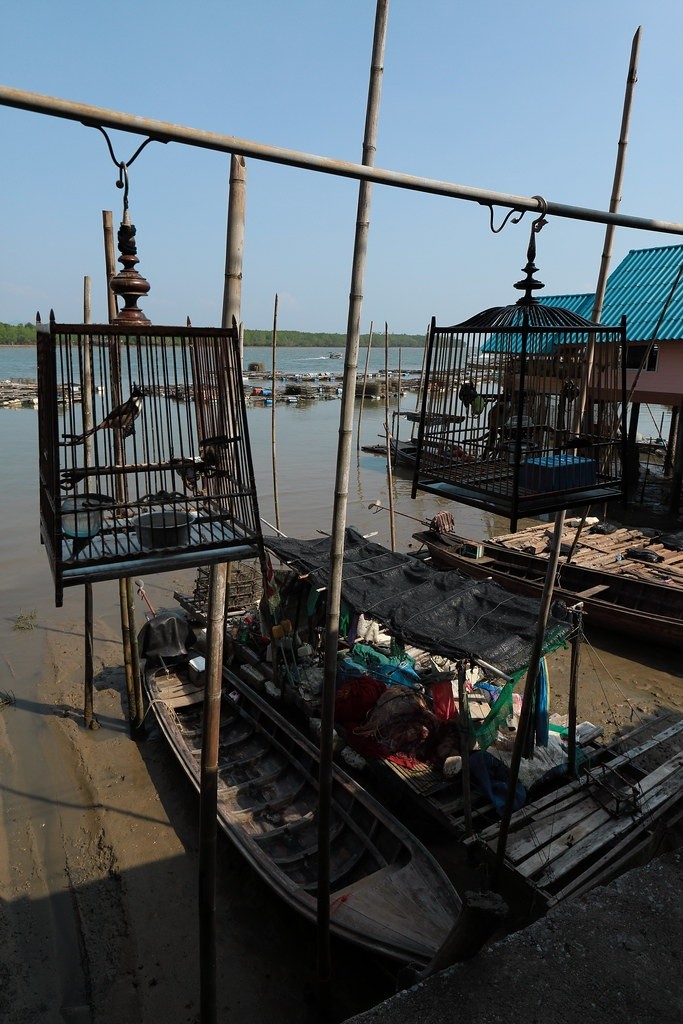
[137,612,465,966]
[411,528,683,635]
[330,353,344,359]
[383,422,465,467]
[581,428,669,447]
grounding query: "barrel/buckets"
[509,443,527,465]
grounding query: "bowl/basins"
[131,511,195,548]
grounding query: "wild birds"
[459,382,484,415]
[75,389,147,443]
[198,435,241,462]
[486,394,511,454]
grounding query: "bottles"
[249,632,261,649]
[616,549,622,561]
[232,619,239,639]
[240,621,248,641]
